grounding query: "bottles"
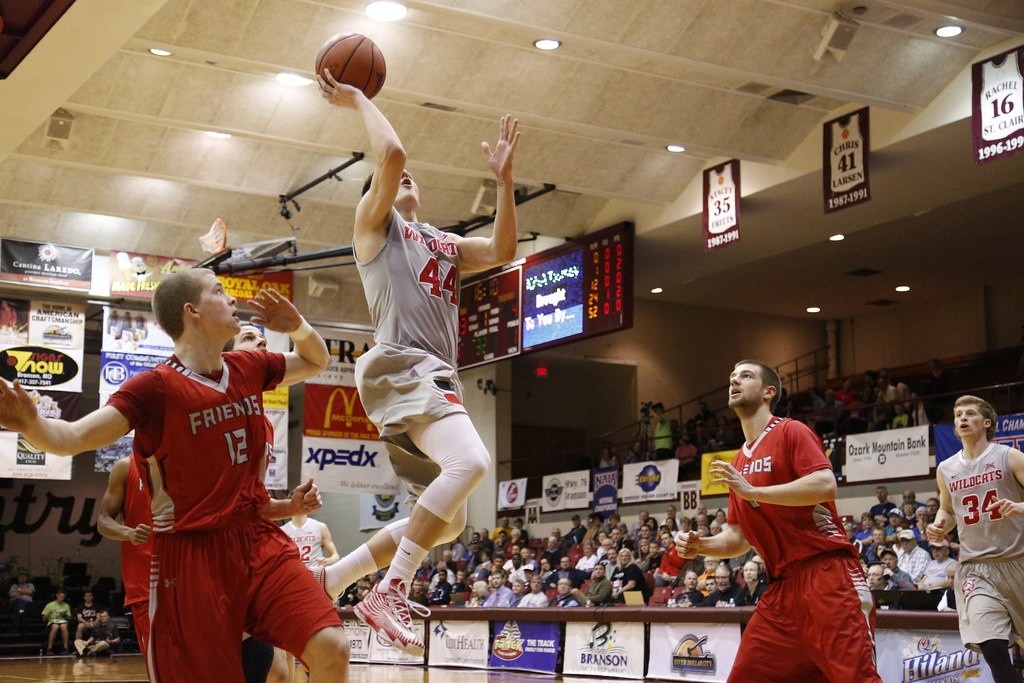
[465,601,469,607]
[668,599,676,608]
[586,600,591,607]
[729,598,735,607]
[506,601,510,607]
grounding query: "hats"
[887,508,904,519]
[17,571,28,578]
[899,529,915,540]
[523,564,537,572]
[881,548,897,558]
[927,539,949,548]
[552,527,561,534]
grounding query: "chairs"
[91,585,113,611]
[27,592,51,619]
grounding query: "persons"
[926,395,1024,683]
[304,64,520,656]
[0,268,353,683]
[0,360,1024,659]
[672,359,884,683]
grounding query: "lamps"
[41,107,76,153]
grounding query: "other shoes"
[75,653,80,657]
[60,648,69,655]
[46,649,54,655]
[18,612,25,617]
[86,650,92,656]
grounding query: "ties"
[491,593,501,608]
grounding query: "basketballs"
[314,34,387,102]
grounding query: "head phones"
[741,561,766,580]
[726,564,735,585]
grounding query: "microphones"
[752,578,758,581]
[560,593,573,601]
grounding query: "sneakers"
[353,577,432,657]
[307,565,346,606]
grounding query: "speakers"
[815,19,858,65]
[42,115,75,151]
[471,179,498,217]
[308,279,338,300]
